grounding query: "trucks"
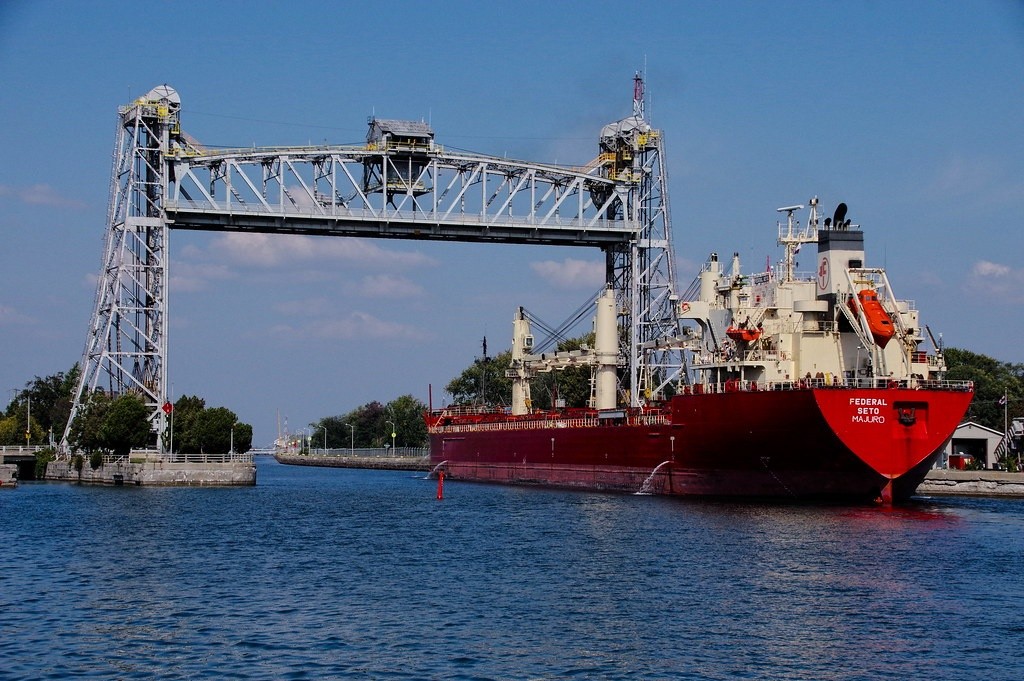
[949,454,975,471]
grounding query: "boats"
[0,464,19,487]
[422,193,975,507]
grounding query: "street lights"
[345,423,354,456]
[319,426,328,457]
[303,427,311,456]
[298,428,305,454]
[385,420,397,457]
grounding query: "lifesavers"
[967,383,972,392]
[888,381,897,390]
[682,303,690,311]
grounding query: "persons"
[889,370,924,388]
[792,372,840,390]
